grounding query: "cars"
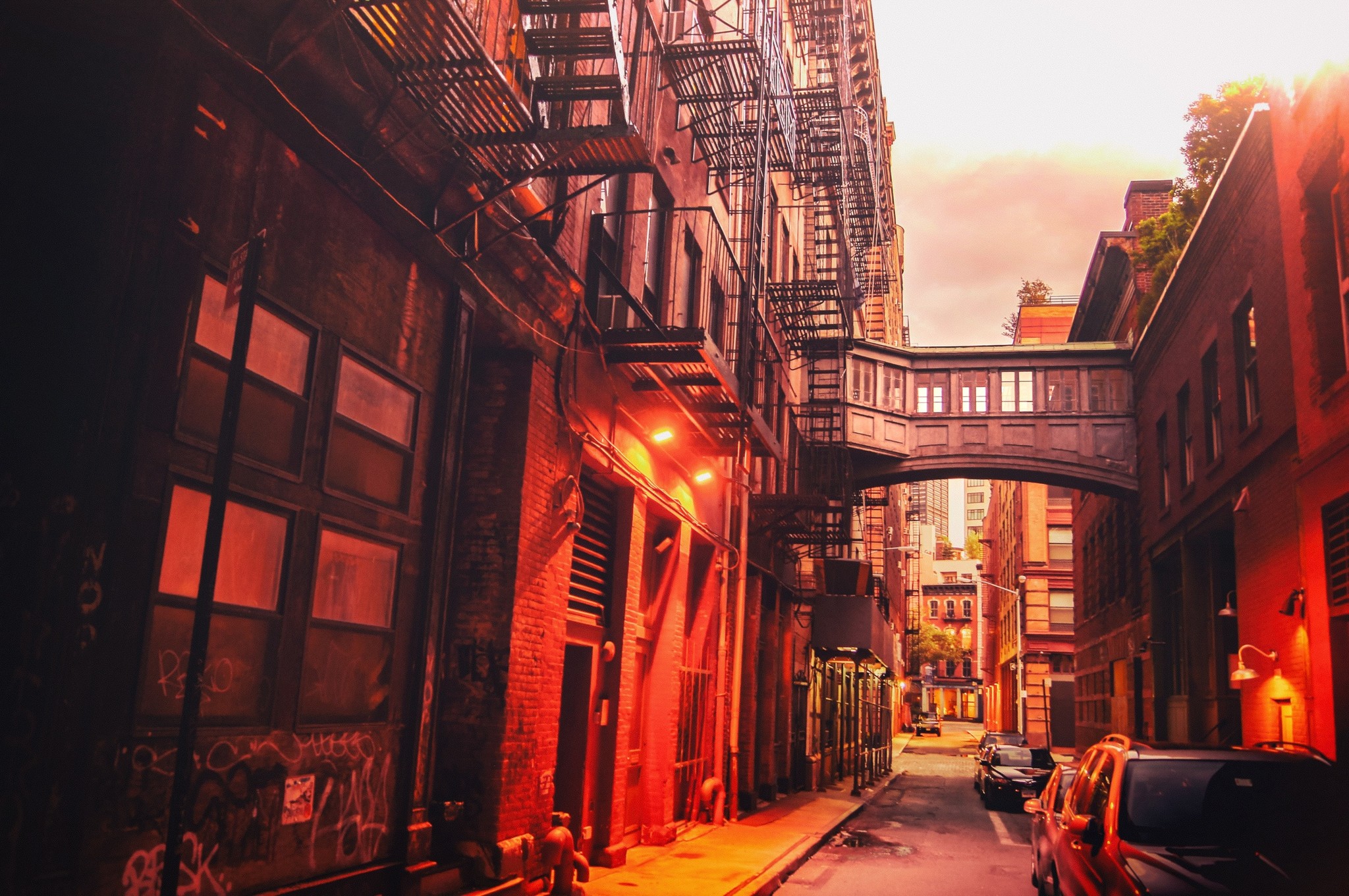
[915,712,942,737]
[975,729,1063,812]
[1023,760,1082,896]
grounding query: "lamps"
[1219,589,1261,617]
[976,563,983,574]
[1139,640,1149,653]
[1230,645,1279,691]
[1280,587,1304,617]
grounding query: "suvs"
[1037,733,1349,896]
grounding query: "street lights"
[956,576,1023,734]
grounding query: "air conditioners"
[888,526,894,535]
[661,10,704,71]
[898,561,902,569]
[501,142,553,222]
[890,622,896,631]
[898,302,901,312]
[902,488,907,495]
[598,294,654,333]
[904,527,910,536]
[901,570,906,577]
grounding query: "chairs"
[1000,752,1012,766]
[1008,737,1017,744]
[987,737,998,744]
